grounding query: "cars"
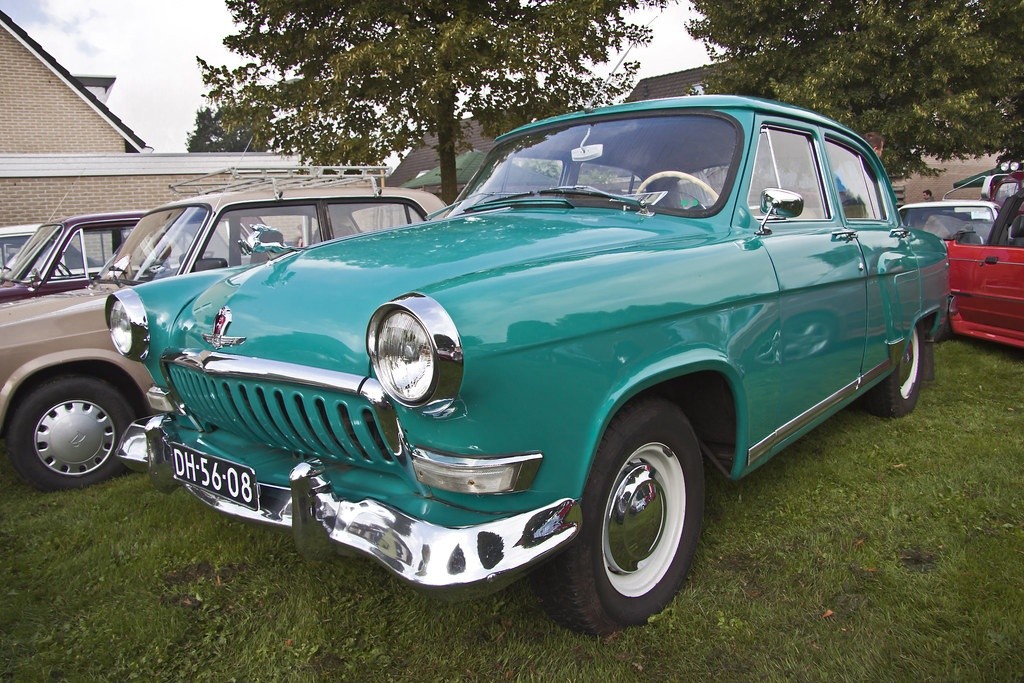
[0,166,455,491]
[896,161,1024,348]
[0,224,130,287]
[0,210,269,306]
[105,95,949,638]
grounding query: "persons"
[923,189,934,201]
